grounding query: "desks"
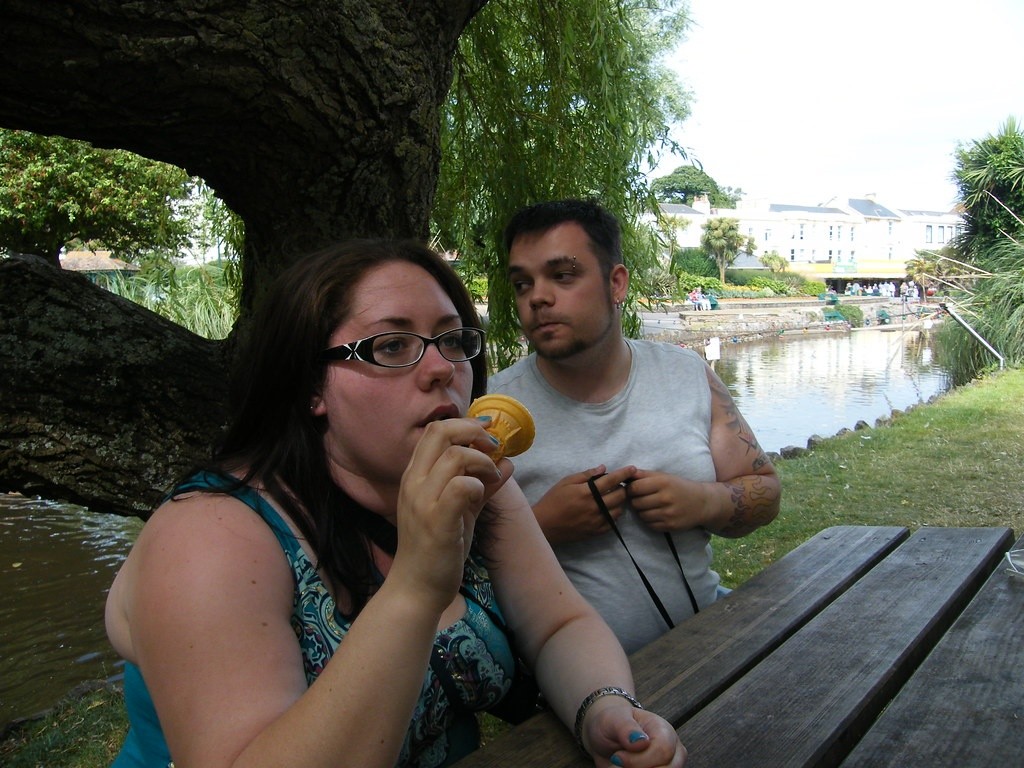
[449,526,1024,768]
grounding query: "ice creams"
[464,394,536,477]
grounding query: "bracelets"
[575,687,643,758]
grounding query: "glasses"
[324,328,486,368]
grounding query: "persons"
[846,282,896,297]
[900,283,920,298]
[688,286,711,312]
[104,240,689,768]
[484,200,782,653]
